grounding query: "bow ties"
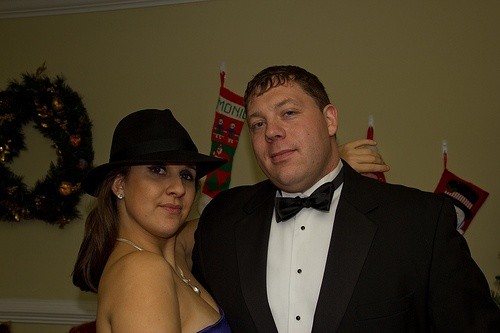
[273,182,335,223]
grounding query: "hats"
[80,108,229,198]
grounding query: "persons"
[72,108,389,333]
[193,64,500,333]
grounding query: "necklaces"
[115,237,202,295]
